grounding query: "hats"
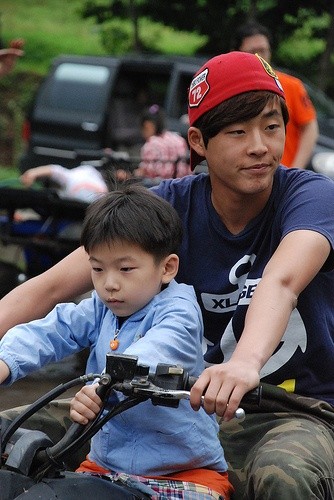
[187,51,285,173]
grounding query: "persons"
[0,185,235,500]
[0,52,334,500]
[228,22,318,170]
[113,104,193,181]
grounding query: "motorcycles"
[0,343,263,500]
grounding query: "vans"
[18,53,334,205]
